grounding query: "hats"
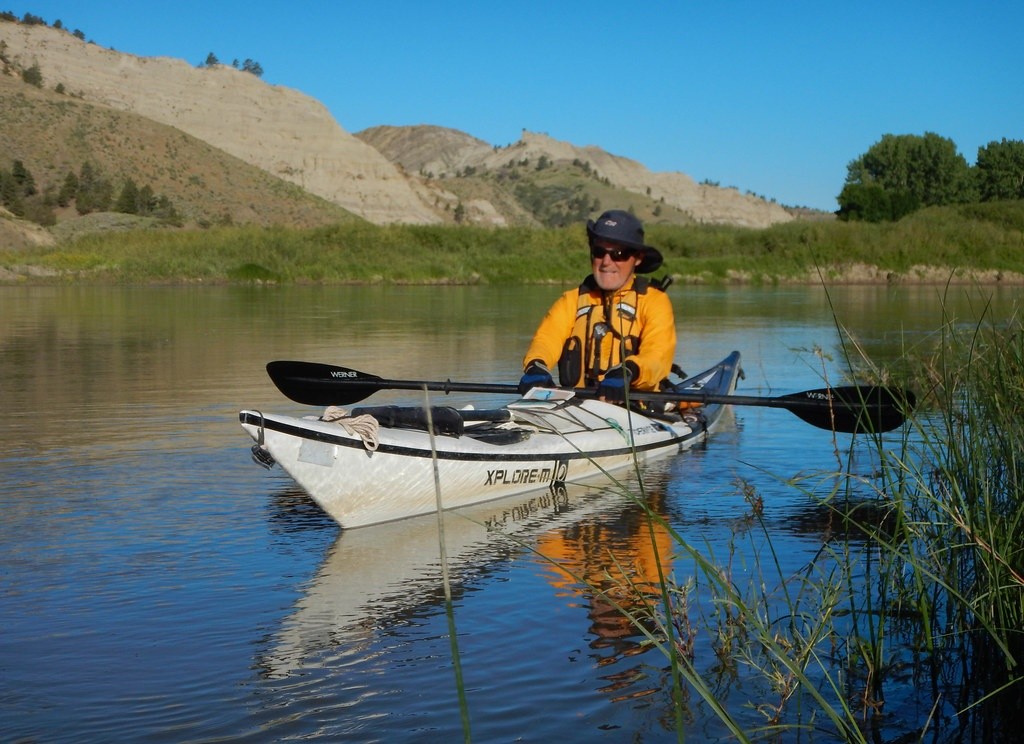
[586,211,663,274]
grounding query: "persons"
[519,210,677,409]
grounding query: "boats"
[235,351,742,534]
[254,460,670,677]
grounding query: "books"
[506,387,575,410]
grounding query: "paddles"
[265,358,919,459]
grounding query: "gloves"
[599,366,633,400]
[519,364,555,396]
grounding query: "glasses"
[591,245,634,262]
